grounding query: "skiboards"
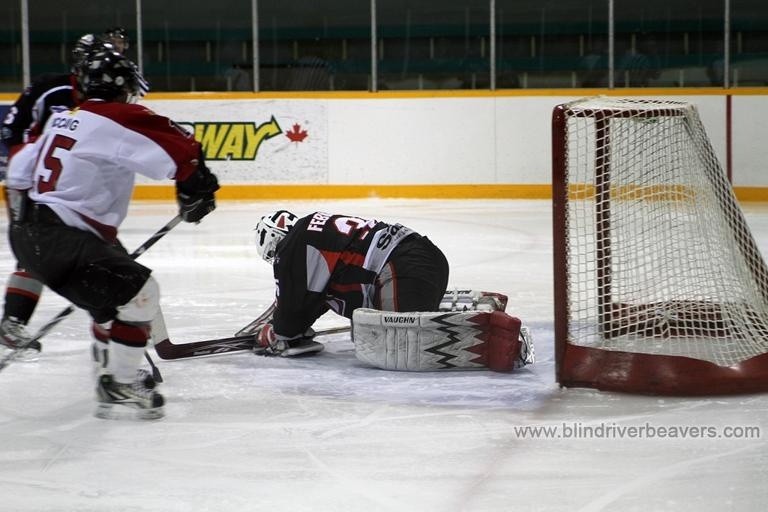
[149,305,350,360]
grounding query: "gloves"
[255,323,280,349]
[175,150,223,225]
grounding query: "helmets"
[254,209,300,266]
[65,26,150,105]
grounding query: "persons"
[0,32,116,353]
[5,52,221,411]
[106,26,130,56]
[250,206,532,377]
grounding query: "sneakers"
[0,316,41,350]
[96,365,165,408]
